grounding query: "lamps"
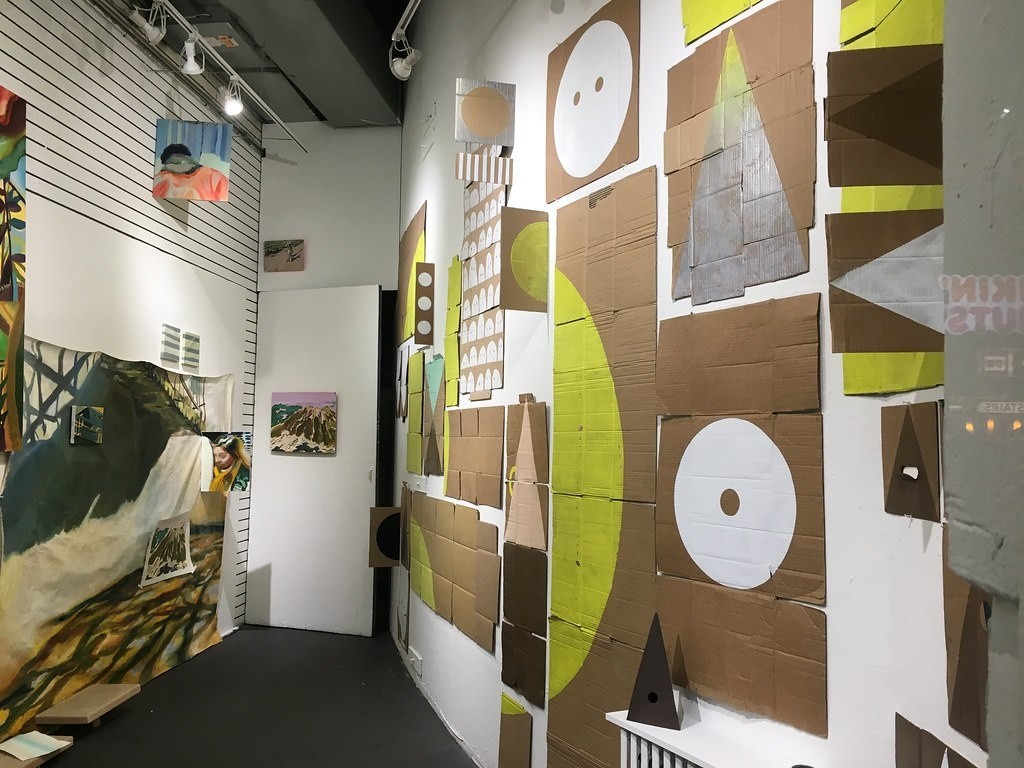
[224,89,244,115]
[129,10,166,46]
[180,39,202,75]
[389,27,422,82]
